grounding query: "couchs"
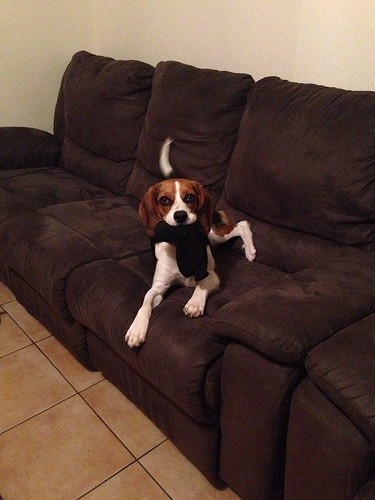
[0,49,375,500]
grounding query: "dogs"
[125,138,258,348]
